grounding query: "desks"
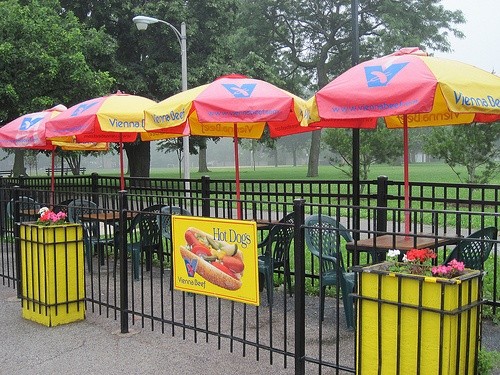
[346,234,463,261]
[79,211,138,266]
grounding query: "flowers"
[385,248,465,278]
[37,208,67,224]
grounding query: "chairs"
[441,226,498,269]
[258,212,356,332]
[7,196,104,273]
[113,205,191,290]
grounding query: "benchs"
[46,167,87,176]
[0,169,14,177]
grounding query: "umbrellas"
[0,103,110,211]
[45,90,190,190]
[145,73,311,220]
[300,47,500,240]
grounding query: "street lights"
[133,15,191,212]
